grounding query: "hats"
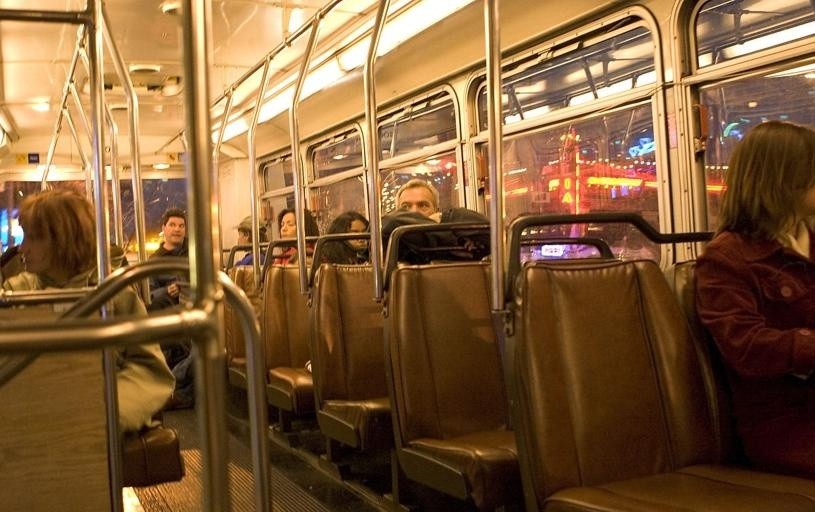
[237,216,266,231]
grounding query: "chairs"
[0,257,815,512]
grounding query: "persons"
[141,207,190,367]
[318,211,371,264]
[223,214,267,274]
[692,122,815,478]
[271,206,320,267]
[0,187,177,432]
[381,177,492,263]
